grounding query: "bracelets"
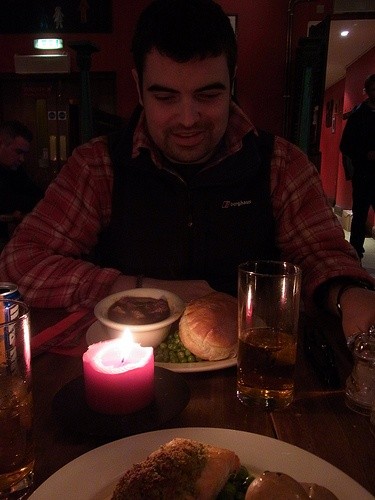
[136,274,143,289]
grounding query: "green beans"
[153,327,196,362]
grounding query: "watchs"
[336,281,375,315]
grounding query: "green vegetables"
[214,463,257,500]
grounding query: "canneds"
[0,282,23,379]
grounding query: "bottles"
[345,327,375,414]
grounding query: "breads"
[179,291,251,360]
[112,437,242,500]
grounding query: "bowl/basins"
[95,288,184,347]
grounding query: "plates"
[26,428,375,500]
[86,322,238,372]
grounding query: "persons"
[339,73,375,261]
[0,0,375,354]
[0,120,44,254]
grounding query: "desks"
[1,297,375,498]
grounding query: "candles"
[82,327,154,415]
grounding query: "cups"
[235,261,302,410]
[0,299,36,498]
